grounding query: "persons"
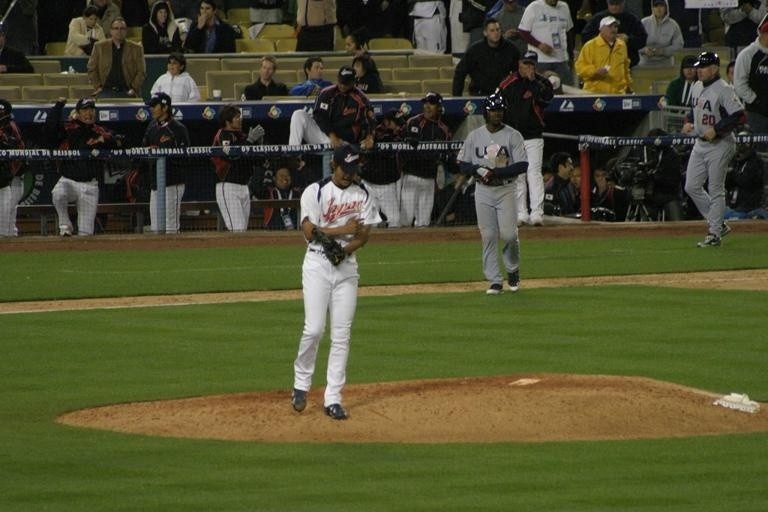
[449,0,768,249]
[456,94,529,294]
[2,0,488,238]
[292,145,376,419]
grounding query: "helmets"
[694,51,720,67]
[483,95,510,118]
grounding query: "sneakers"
[508,271,519,292]
[325,404,346,419]
[59,225,72,236]
[292,389,307,411]
[487,284,504,294]
[721,222,731,237]
[697,234,722,248]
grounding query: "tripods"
[625,200,653,222]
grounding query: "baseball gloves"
[312,228,346,266]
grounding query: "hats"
[76,99,97,113]
[334,146,360,168]
[338,65,356,85]
[519,50,537,66]
[0,99,13,119]
[600,16,621,29]
[145,93,171,106]
[420,91,443,105]
[385,109,407,127]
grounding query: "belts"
[697,137,707,142]
[310,249,325,254]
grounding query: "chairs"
[574,14,751,98]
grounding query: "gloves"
[247,125,265,144]
[478,166,505,185]
